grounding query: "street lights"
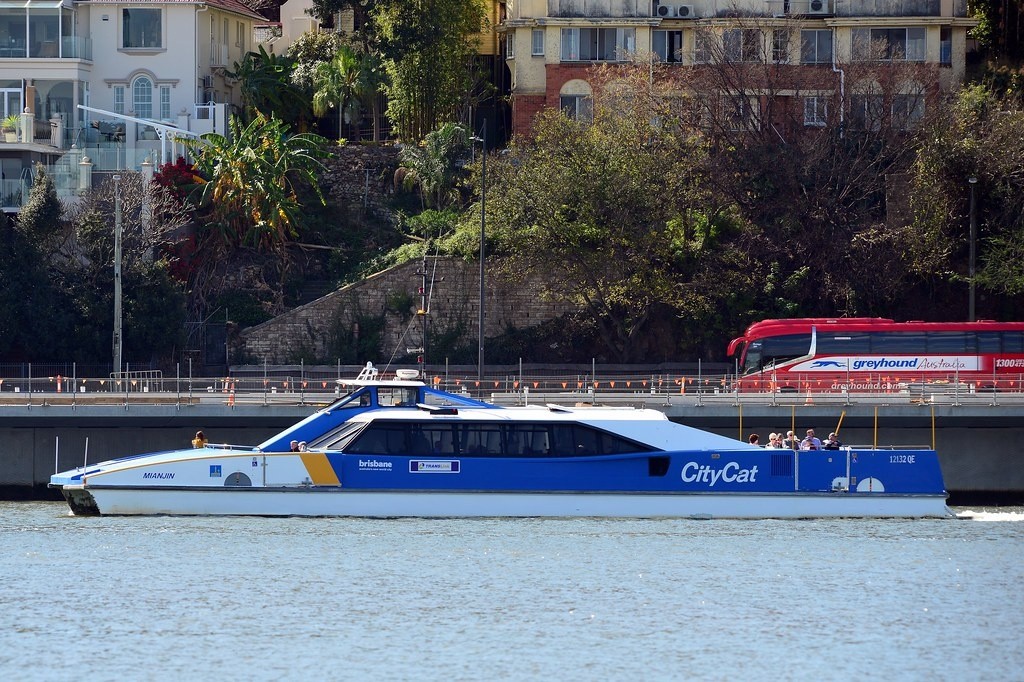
[468,135,484,401]
[963,172,982,319]
[111,172,127,392]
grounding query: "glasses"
[771,438,776,440]
[789,435,792,436]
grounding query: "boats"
[47,235,961,524]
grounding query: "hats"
[805,435,813,442]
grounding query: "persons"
[748,429,842,451]
[289,440,310,452]
[191,430,208,449]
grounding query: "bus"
[723,314,1024,396]
